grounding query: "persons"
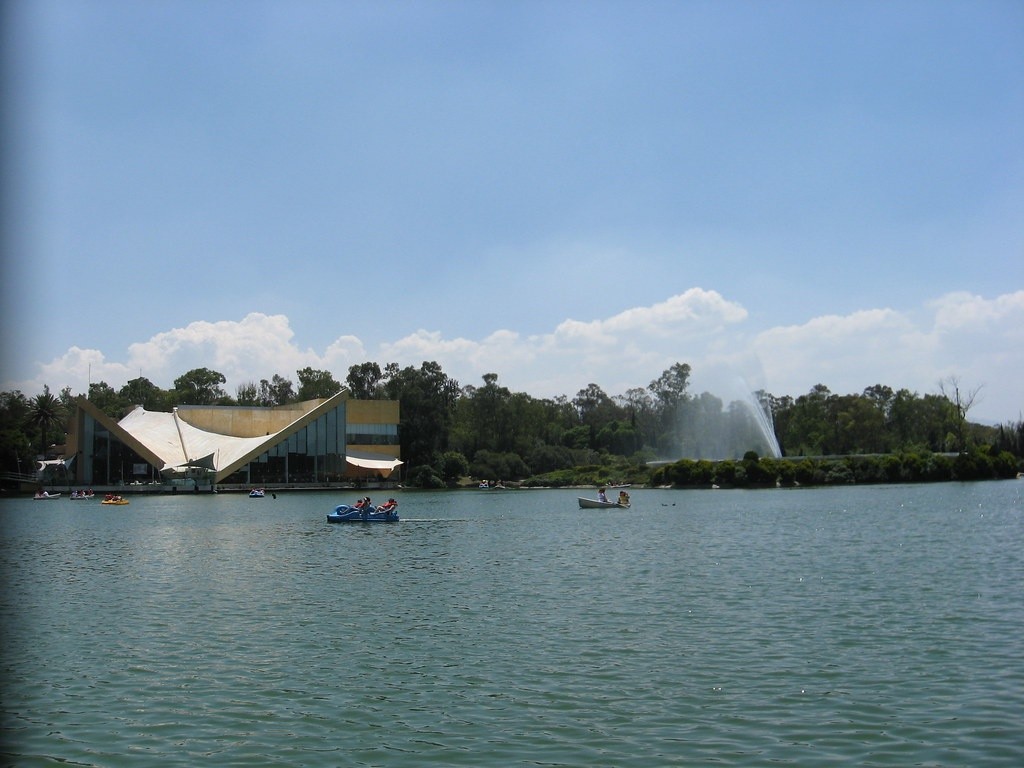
[598,487,607,502]
[113,494,122,501]
[106,492,112,501]
[81,488,86,496]
[72,490,77,498]
[89,487,93,495]
[617,490,630,504]
[340,497,370,514]
[43,489,48,496]
[374,498,397,514]
[356,499,362,508]
[35,490,40,497]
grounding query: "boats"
[479,480,491,488]
[579,488,631,509]
[494,483,506,490]
[34,493,62,499]
[327,501,401,522]
[101,494,129,505]
[71,493,95,500]
[249,489,266,498]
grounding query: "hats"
[388,498,394,502]
